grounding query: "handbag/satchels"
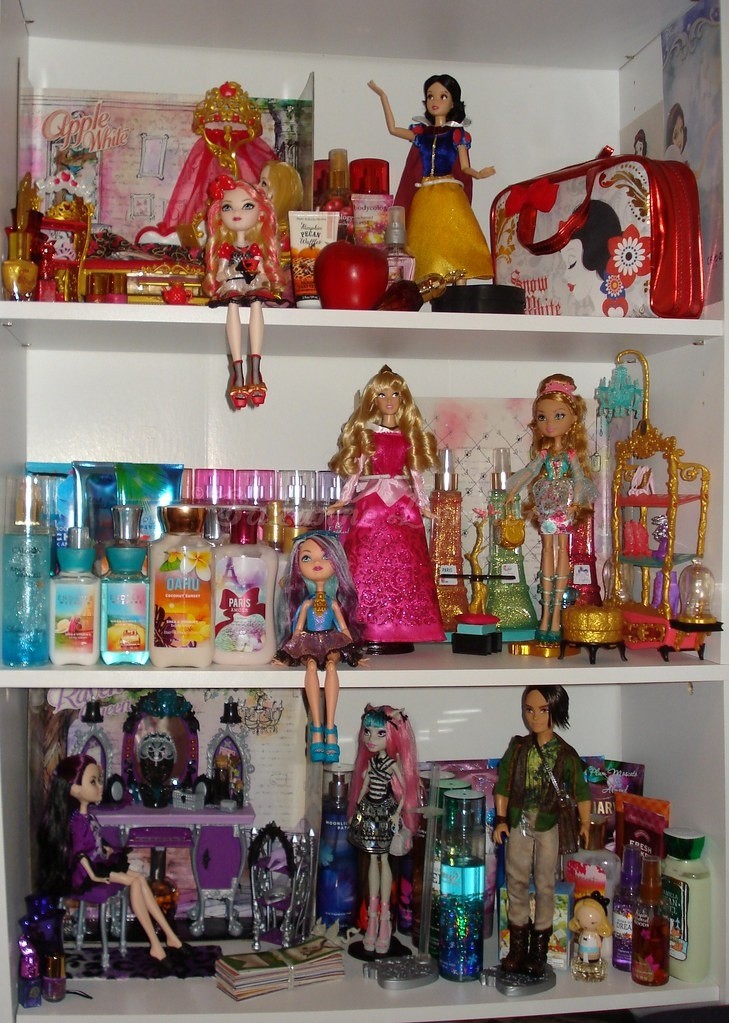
[490,146,704,316]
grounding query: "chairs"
[250,827,316,950]
[57,826,126,971]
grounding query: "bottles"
[319,147,414,287]
[486,448,540,627]
[2,461,343,666]
[426,450,474,629]
[141,847,177,932]
[315,764,708,987]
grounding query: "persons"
[367,74,493,286]
[569,890,611,962]
[502,374,601,643]
[206,174,286,409]
[37,754,197,969]
[256,161,304,307]
[346,702,422,954]
[326,370,447,641]
[271,528,372,766]
[491,684,592,976]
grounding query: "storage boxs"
[499,881,574,970]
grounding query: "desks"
[90,803,256,933]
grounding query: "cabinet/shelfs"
[610,418,711,661]
[2,2,729,1023]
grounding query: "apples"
[314,241,389,310]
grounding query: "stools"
[558,606,628,666]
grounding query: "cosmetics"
[2,461,344,667]
[316,759,669,985]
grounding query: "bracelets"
[495,816,507,824]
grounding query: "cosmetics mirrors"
[111,781,124,802]
